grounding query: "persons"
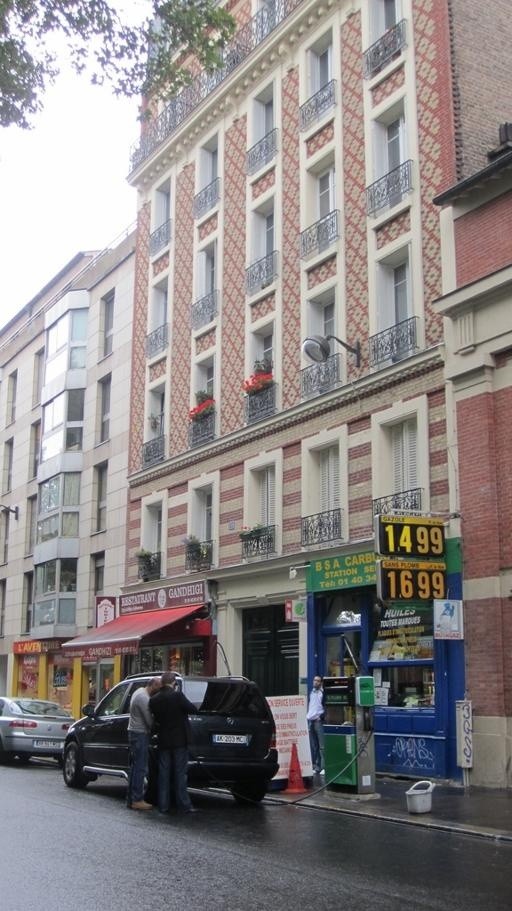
[126,677,163,810]
[149,673,202,814]
[306,674,327,776]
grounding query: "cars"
[0,697,76,766]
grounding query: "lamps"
[289,563,312,579]
[301,333,360,367]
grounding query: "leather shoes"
[132,801,153,811]
[184,804,201,813]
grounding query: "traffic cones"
[281,742,307,794]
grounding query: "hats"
[161,673,178,685]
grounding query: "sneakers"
[313,768,318,774]
[319,769,325,776]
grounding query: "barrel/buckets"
[405,780,433,814]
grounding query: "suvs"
[61,669,280,810]
